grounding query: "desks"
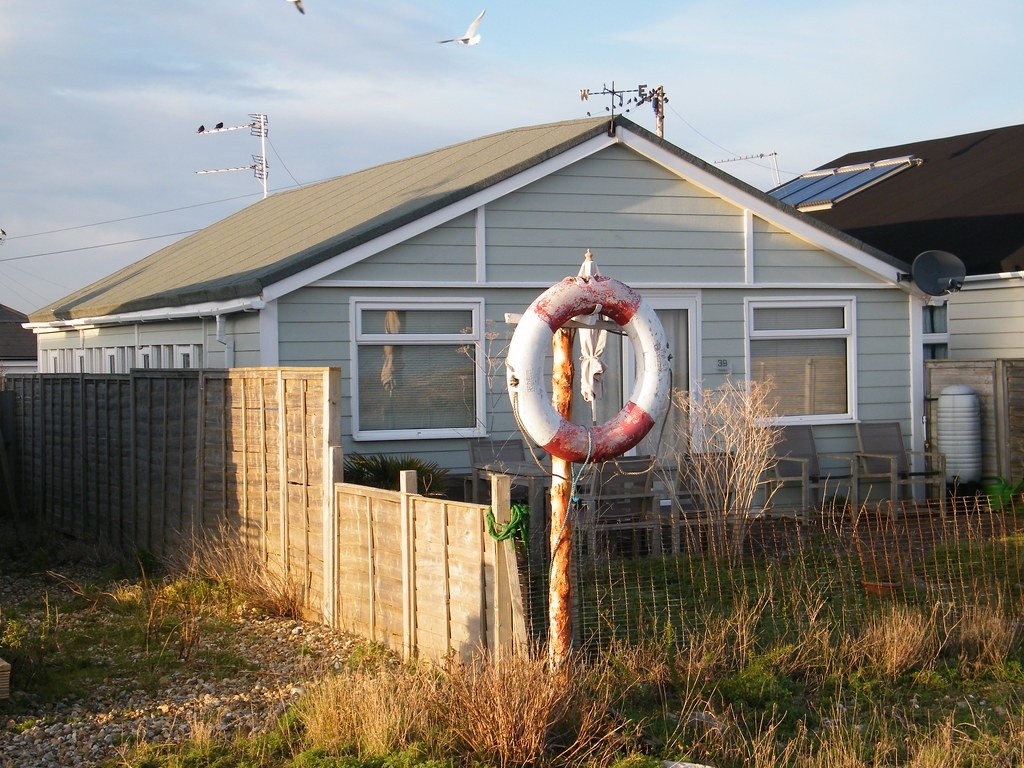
[473,458,677,566]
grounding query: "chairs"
[765,424,858,525]
[647,452,733,558]
[463,437,529,508]
[576,455,656,566]
[852,422,948,520]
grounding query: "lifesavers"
[504,272,674,465]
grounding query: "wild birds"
[287,1,305,14]
[438,9,486,46]
[578,89,669,120]
[215,122,224,129]
[197,125,204,134]
